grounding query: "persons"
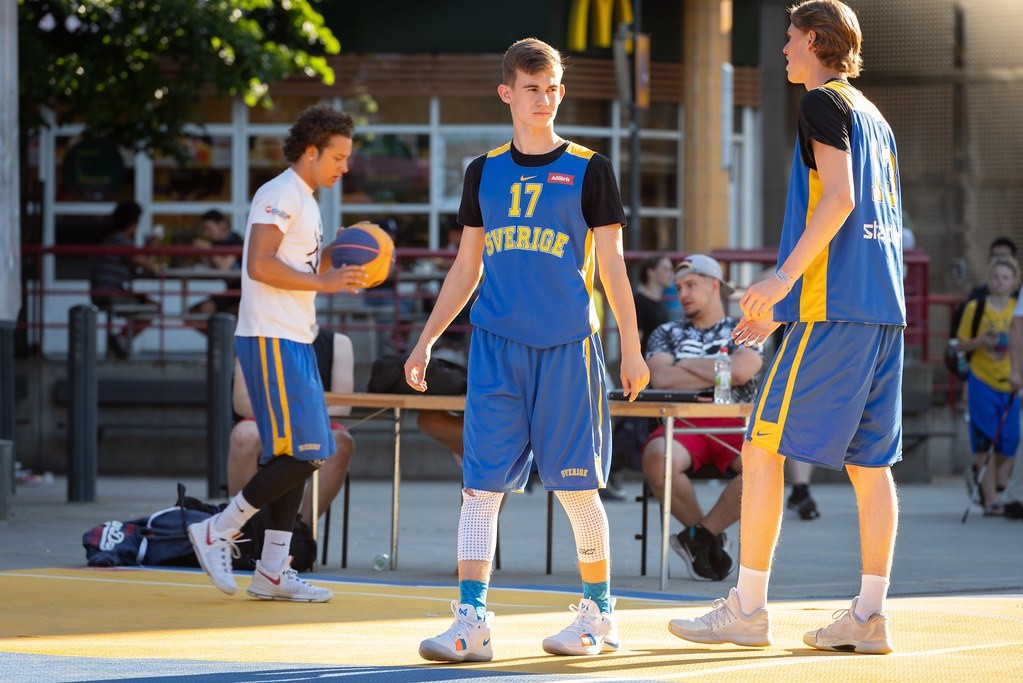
[92,203,162,362]
[947,237,1023,514]
[189,211,243,336]
[789,458,818,518]
[404,38,649,662]
[645,253,764,582]
[417,221,485,464]
[600,255,673,500]
[225,328,354,525]
[186,104,367,602]
[667,0,907,654]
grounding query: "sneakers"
[710,531,735,580]
[247,555,333,604]
[543,596,617,655]
[670,526,718,582]
[188,512,251,595]
[419,599,494,662]
[803,596,892,653]
[668,586,769,647]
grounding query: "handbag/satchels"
[175,483,316,574]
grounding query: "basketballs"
[330,223,396,289]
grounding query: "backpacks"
[944,295,986,380]
[82,506,214,567]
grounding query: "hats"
[676,253,735,299]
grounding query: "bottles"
[712,347,732,406]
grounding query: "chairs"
[634,385,726,577]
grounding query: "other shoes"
[786,491,819,519]
[599,468,628,499]
[984,498,1006,517]
[963,463,980,505]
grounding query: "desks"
[134,270,233,321]
[324,392,754,590]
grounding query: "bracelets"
[775,270,793,288]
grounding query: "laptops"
[609,388,698,402]
